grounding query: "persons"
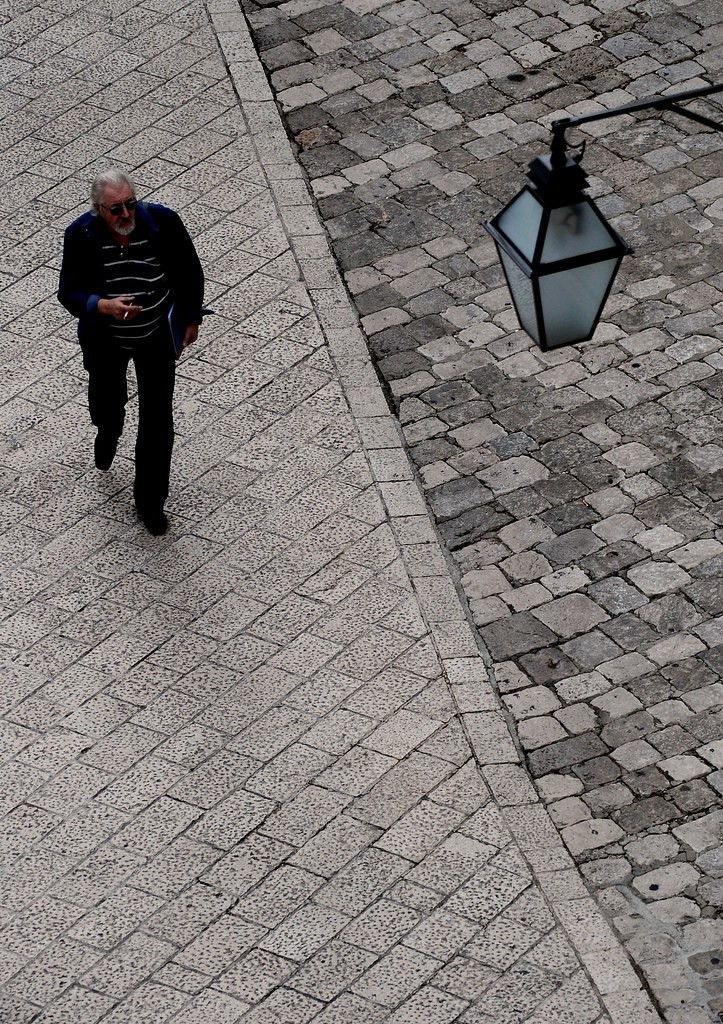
[58,169,205,537]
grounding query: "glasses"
[99,194,138,215]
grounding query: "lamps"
[479,84,723,352]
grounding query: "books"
[160,299,186,360]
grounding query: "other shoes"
[92,425,116,470]
[133,490,169,535]
